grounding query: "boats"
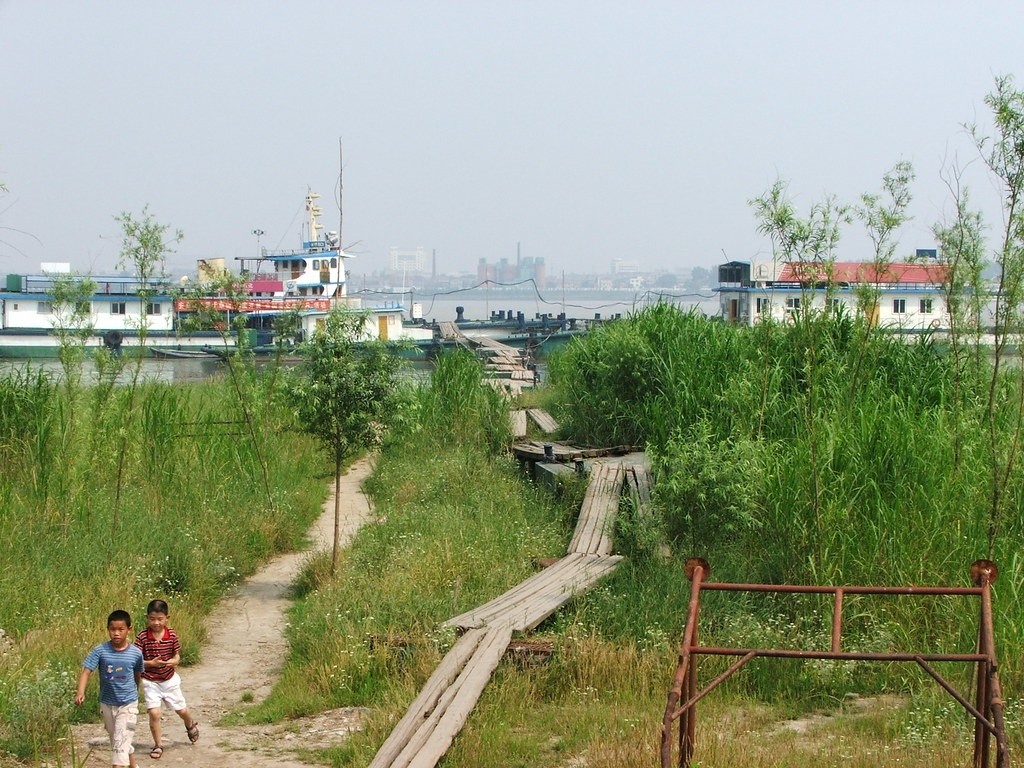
[150,346,221,358]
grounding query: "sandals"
[186,721,199,743]
[150,746,163,759]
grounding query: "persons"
[74,609,145,768]
[133,599,199,759]
